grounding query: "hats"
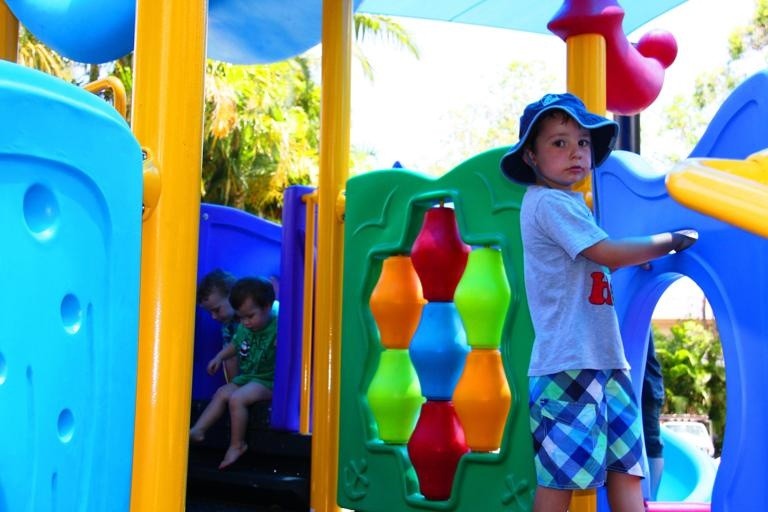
[499,93,620,186]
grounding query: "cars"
[660,421,716,458]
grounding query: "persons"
[188,276,279,468]
[642,330,663,504]
[500,94,684,512]
[196,267,280,383]
